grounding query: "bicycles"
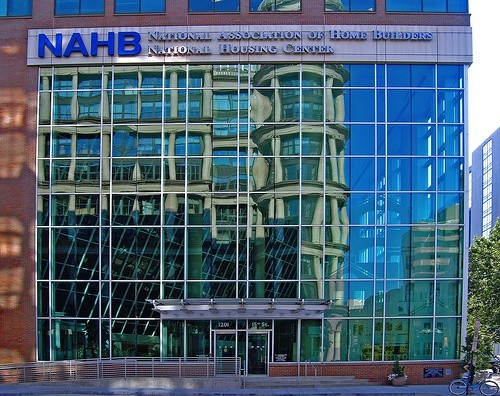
[448,371,499,396]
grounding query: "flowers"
[387,359,408,381]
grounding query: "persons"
[462,357,477,396]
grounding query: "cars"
[482,352,500,374]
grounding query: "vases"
[391,377,406,386]
